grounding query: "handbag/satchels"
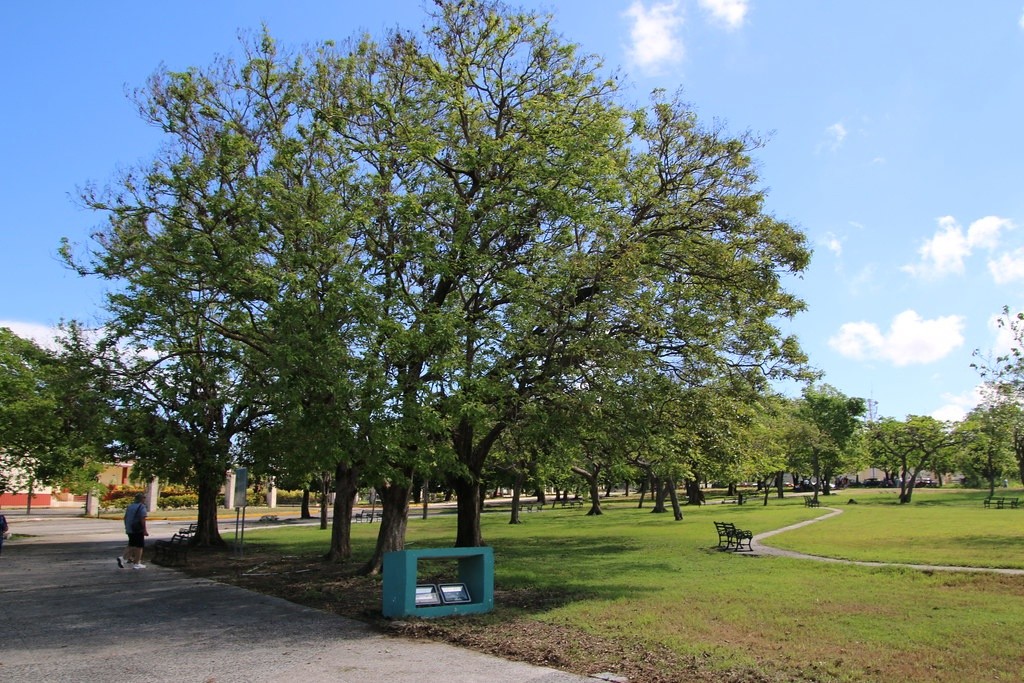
[131,521,143,535]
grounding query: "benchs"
[517,500,543,512]
[561,499,583,508]
[179,524,198,538]
[722,491,758,505]
[983,496,1018,509]
[803,496,820,508]
[151,533,191,568]
[714,521,754,553]
[354,509,383,522]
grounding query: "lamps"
[415,583,441,605]
[437,583,471,604]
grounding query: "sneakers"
[117,556,125,568]
[132,564,146,569]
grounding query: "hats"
[135,493,141,503]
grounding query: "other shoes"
[128,560,133,562]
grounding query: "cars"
[907,476,937,486]
[782,481,794,487]
[712,482,729,488]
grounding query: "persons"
[117,492,148,569]
[1003,479,1008,487]
[795,479,809,491]
[0,514,8,556]
[888,476,910,487]
[834,476,848,489]
